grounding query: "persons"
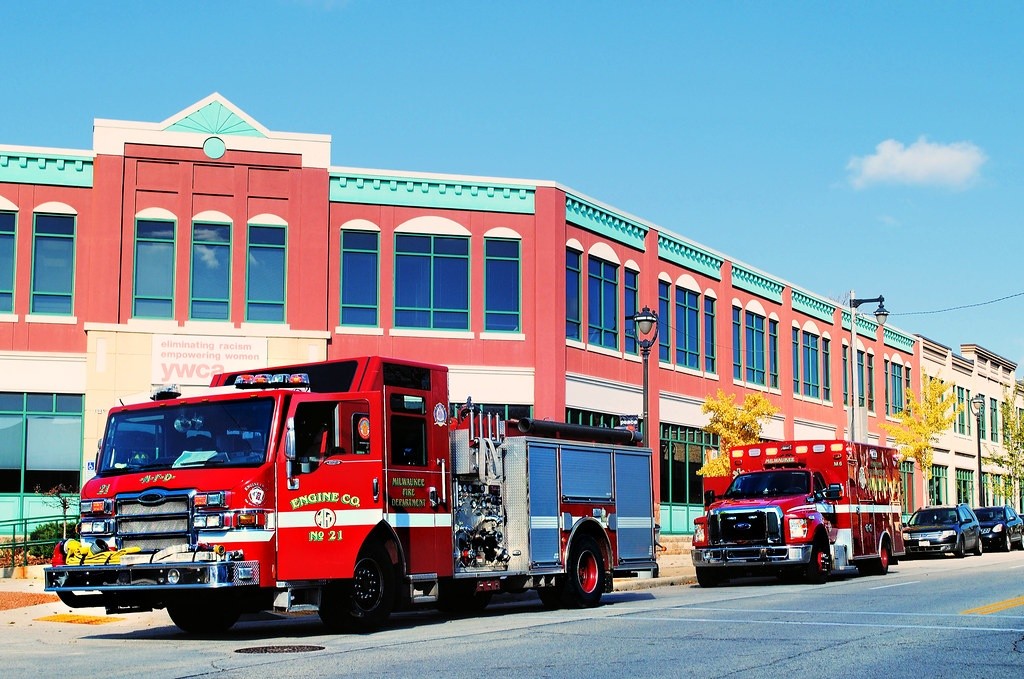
[992,512,1002,520]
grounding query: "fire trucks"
[45,356,659,633]
[688,440,906,587]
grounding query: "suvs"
[969,505,1024,551]
[898,504,984,557]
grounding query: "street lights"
[632,307,660,447]
[847,294,892,440]
[969,396,986,507]
